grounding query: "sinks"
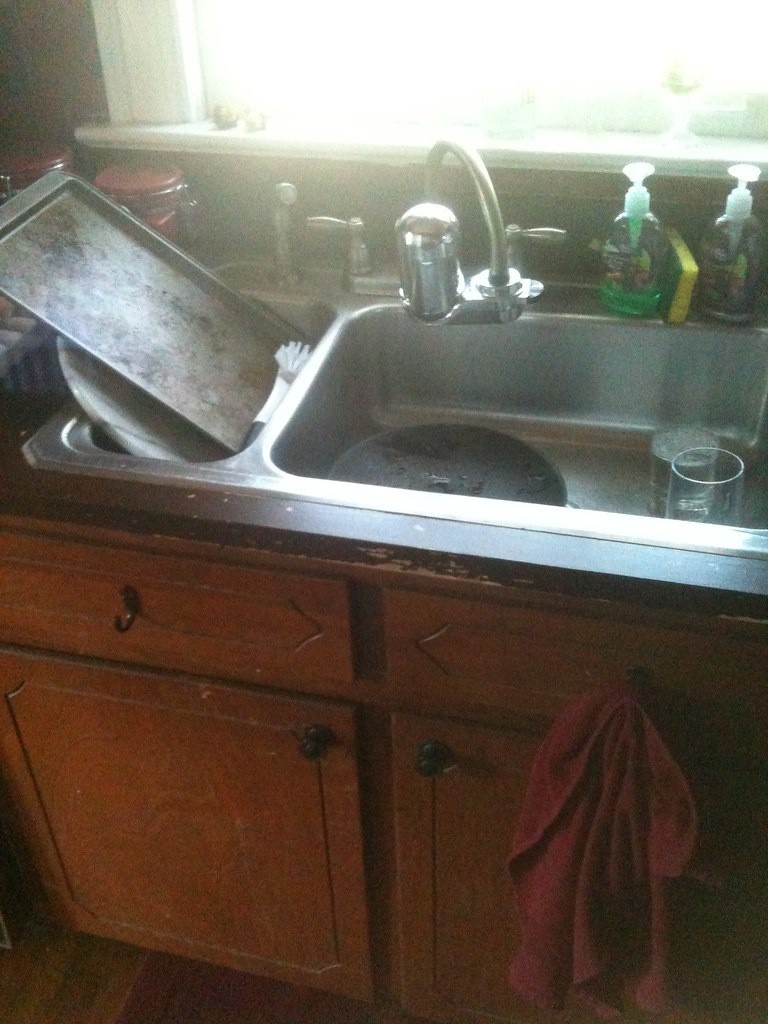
[26,250,353,483]
[246,290,768,554]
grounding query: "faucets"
[306,135,568,325]
[266,183,303,277]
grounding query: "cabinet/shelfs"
[1,519,768,1024]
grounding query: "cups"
[650,430,718,520]
[666,447,744,528]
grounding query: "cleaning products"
[690,164,768,325]
[587,158,672,315]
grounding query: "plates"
[56,332,229,463]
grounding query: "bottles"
[0,143,197,254]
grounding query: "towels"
[503,678,717,1024]
